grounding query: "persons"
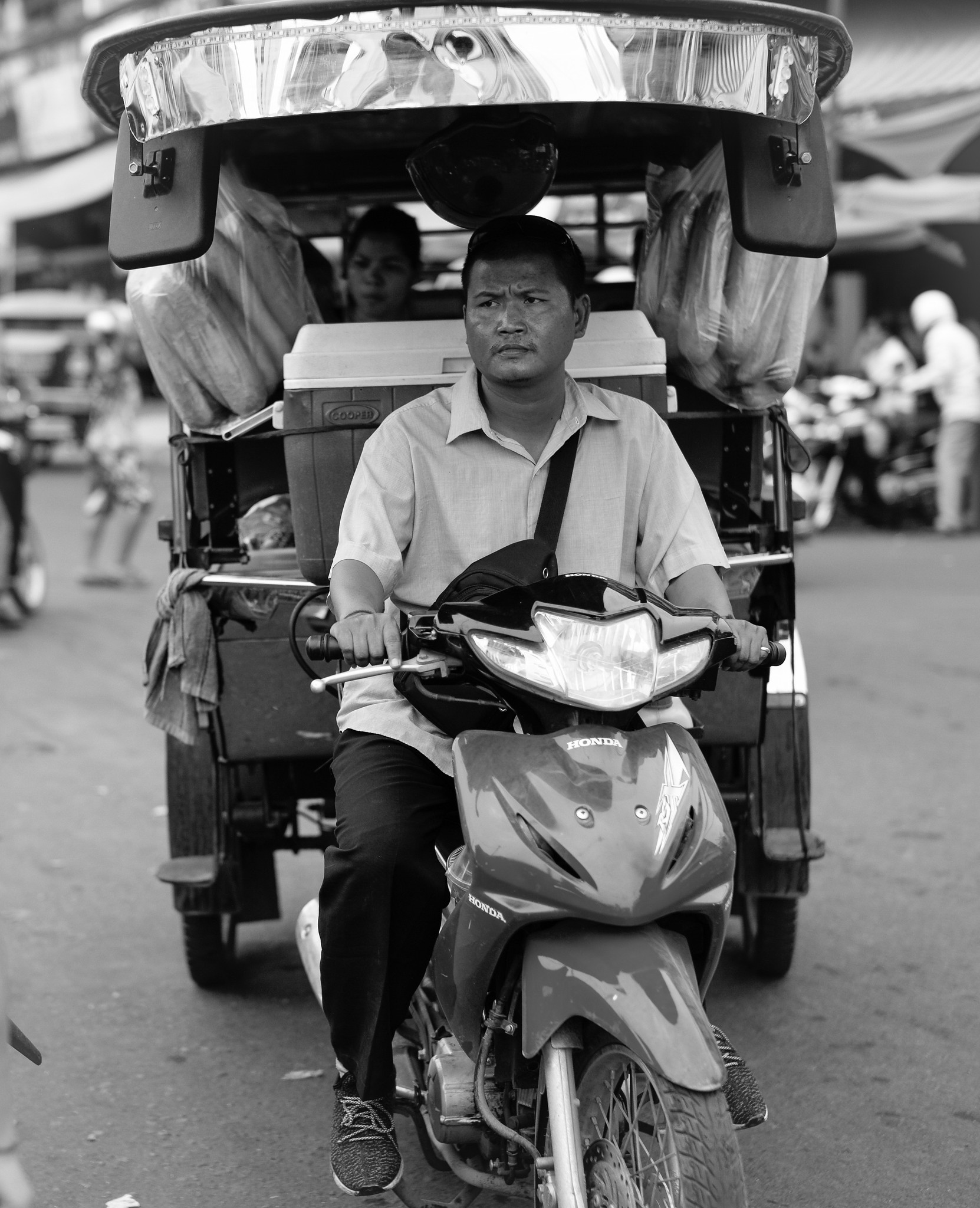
[339,202,424,321]
[81,306,154,584]
[319,215,771,1197]
[854,289,980,533]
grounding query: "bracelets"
[343,609,374,618]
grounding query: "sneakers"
[331,1072,404,1195]
[713,1024,768,1129]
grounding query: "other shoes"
[86,569,148,591]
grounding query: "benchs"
[170,406,786,762]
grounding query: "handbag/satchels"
[393,538,569,739]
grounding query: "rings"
[761,647,771,654]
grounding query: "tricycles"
[76,0,860,1206]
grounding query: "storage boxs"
[284,309,669,584]
